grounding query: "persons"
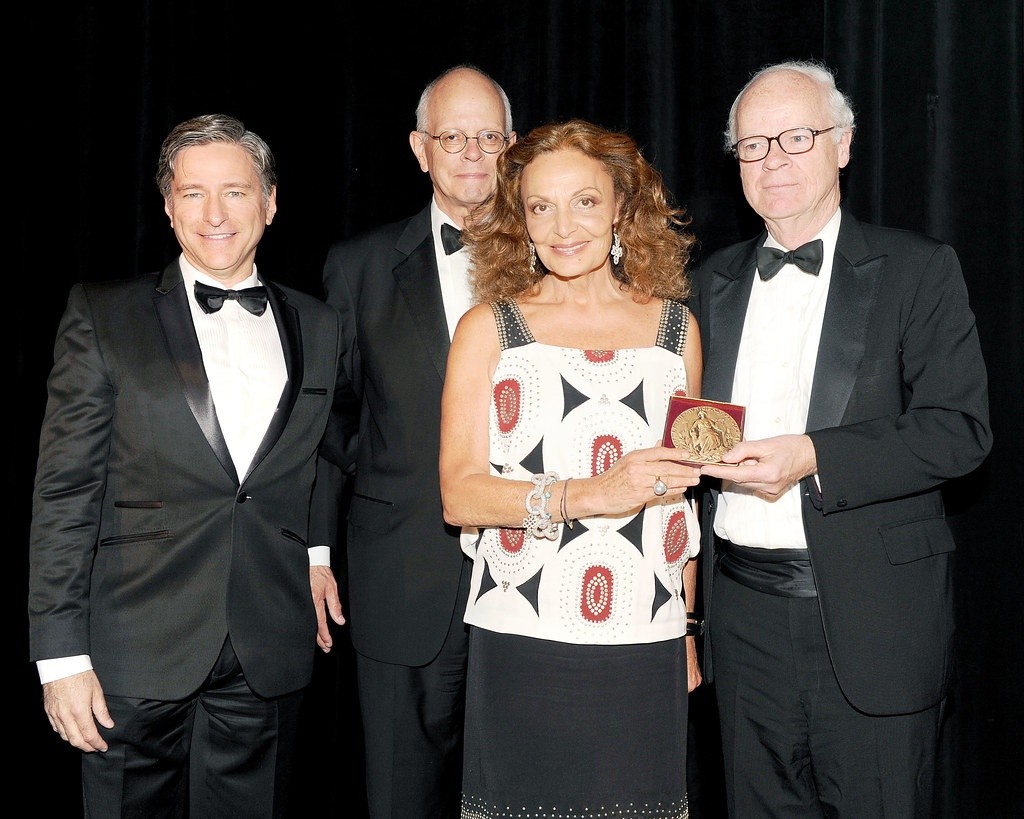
[307,63,549,819]
[678,60,992,819]
[438,122,707,819]
[28,115,342,819]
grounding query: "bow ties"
[194,281,268,316]
[441,222,489,256]
[755,239,824,280]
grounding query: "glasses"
[422,130,510,155]
[729,126,840,162]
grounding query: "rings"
[653,476,667,496]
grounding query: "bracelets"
[560,478,574,531]
[685,610,700,624]
[684,619,705,636]
[522,471,559,541]
